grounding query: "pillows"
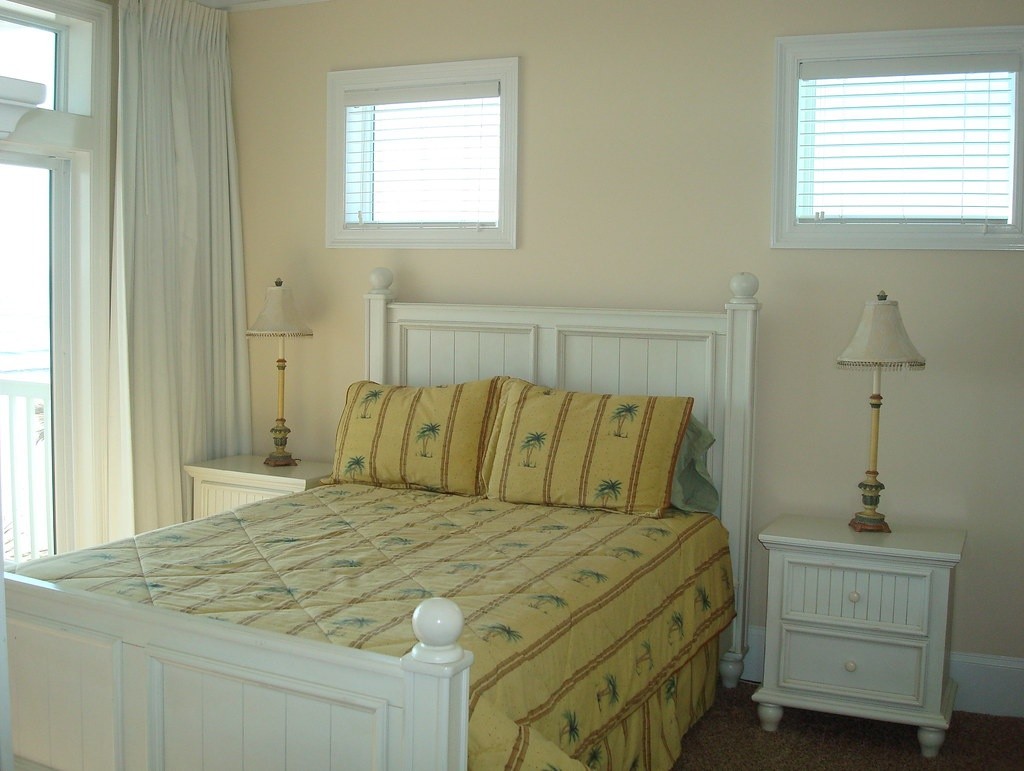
[317,376,513,497]
[487,380,719,515]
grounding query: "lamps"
[245,278,315,468]
[834,290,928,534]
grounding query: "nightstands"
[750,514,968,759]
[182,455,335,522]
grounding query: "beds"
[6,265,763,771]
